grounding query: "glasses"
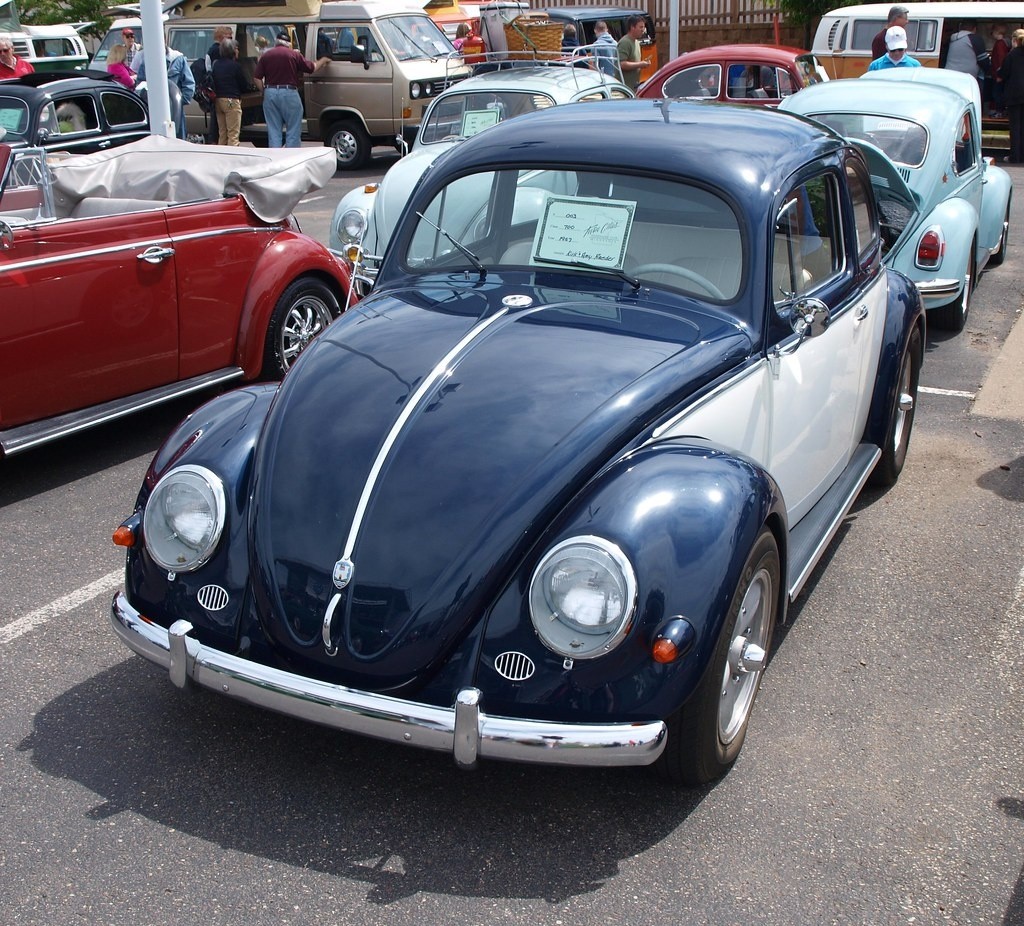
[0,47,12,54]
[224,36,233,40]
[125,35,135,39]
[889,48,904,52]
[235,47,240,51]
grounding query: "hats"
[277,32,291,42]
[122,27,133,34]
[885,26,908,50]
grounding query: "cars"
[329,5,1014,330]
[109,95,928,788]
[0,1,362,459]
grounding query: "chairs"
[662,254,813,301]
[498,241,643,281]
[358,35,369,56]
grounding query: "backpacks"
[189,57,215,114]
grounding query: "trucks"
[159,0,474,171]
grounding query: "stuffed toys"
[51,103,86,133]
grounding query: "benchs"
[498,218,832,285]
[68,194,178,218]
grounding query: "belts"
[265,85,296,90]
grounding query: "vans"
[811,1,1024,153]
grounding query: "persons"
[871,6,909,61]
[944,18,991,103]
[255,34,274,61]
[318,27,355,53]
[591,21,619,79]
[211,38,264,146]
[615,14,651,93]
[736,64,775,89]
[868,25,922,71]
[988,22,1010,119]
[996,28,1024,163]
[454,23,472,50]
[0,37,35,81]
[205,26,233,145]
[560,24,590,69]
[104,28,196,142]
[252,31,332,148]
